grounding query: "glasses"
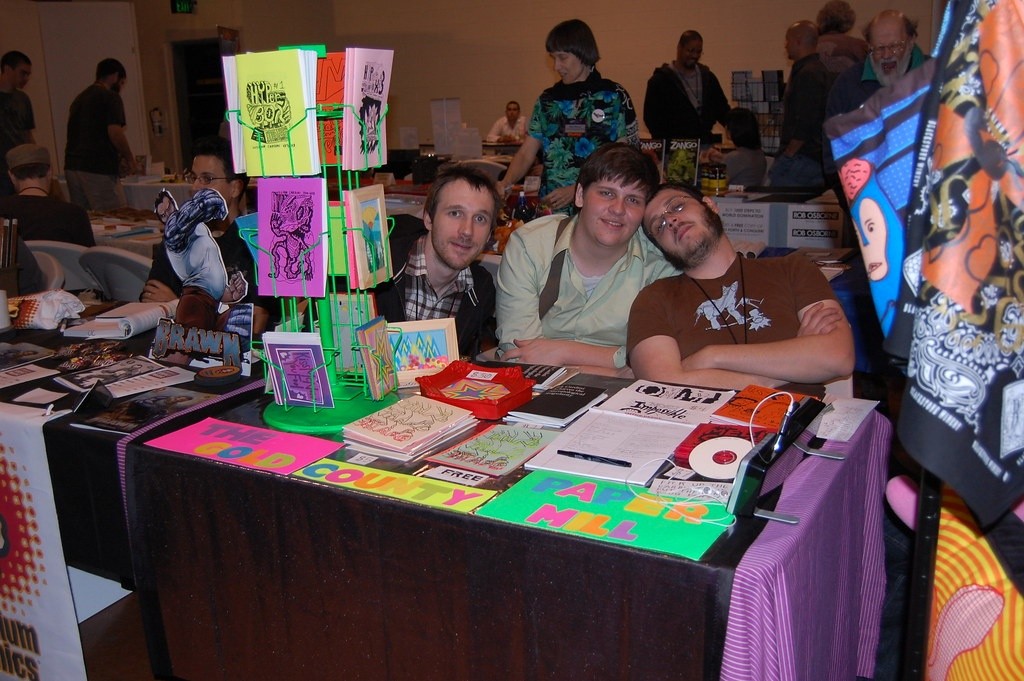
[869,35,911,54]
[185,172,232,186]
[649,195,696,237]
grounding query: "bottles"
[514,191,536,223]
[698,163,727,197]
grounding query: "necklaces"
[17,186,48,195]
[691,253,747,345]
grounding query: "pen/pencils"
[556,450,632,468]
[818,261,845,264]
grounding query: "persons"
[498,149,544,184]
[486,101,528,143]
[767,0,931,194]
[495,19,639,217]
[626,182,856,396]
[496,143,684,378]
[62,56,136,210]
[299,165,499,359]
[643,29,767,186]
[0,50,39,194]
[137,135,281,335]
[0,143,96,248]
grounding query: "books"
[126,153,165,183]
[787,247,853,281]
[87,206,166,244]
[341,357,811,485]
[222,45,395,406]
[640,138,700,186]
[0,300,222,436]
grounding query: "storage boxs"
[713,197,850,250]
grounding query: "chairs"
[78,246,153,303]
[26,251,64,293]
[25,239,99,296]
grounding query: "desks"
[0,140,890,681]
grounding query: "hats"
[6,144,51,169]
